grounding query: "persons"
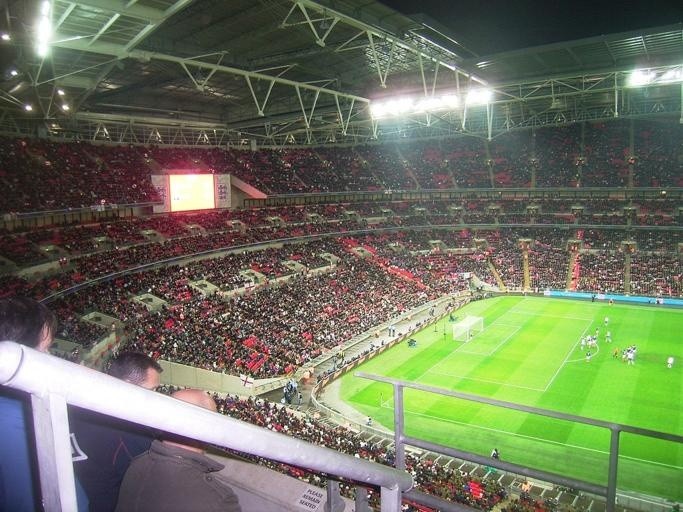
[470,112,683,307]
[331,415,683,512]
[667,356,673,368]
[0,294,332,512]
[0,134,473,356]
[579,316,636,366]
